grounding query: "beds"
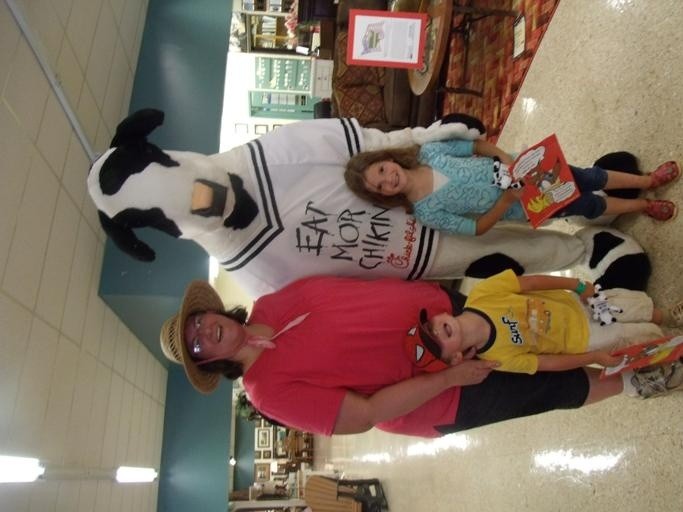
[404,1,517,100]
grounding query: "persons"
[345,138,681,236]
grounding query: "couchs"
[327,1,437,135]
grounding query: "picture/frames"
[258,429,270,448]
[254,419,272,427]
[254,463,271,481]
[254,451,271,459]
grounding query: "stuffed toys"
[87,108,654,301]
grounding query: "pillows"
[333,32,386,124]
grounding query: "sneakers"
[668,301,683,327]
[645,161,681,192]
[630,355,683,400]
[643,199,677,221]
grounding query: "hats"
[403,308,449,373]
[191,311,205,354]
[159,279,224,395]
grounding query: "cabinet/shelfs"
[249,58,333,119]
[243,0,336,57]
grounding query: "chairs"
[303,474,387,512]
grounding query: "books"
[508,135,579,229]
[243,0,282,49]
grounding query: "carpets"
[426,1,558,146]
